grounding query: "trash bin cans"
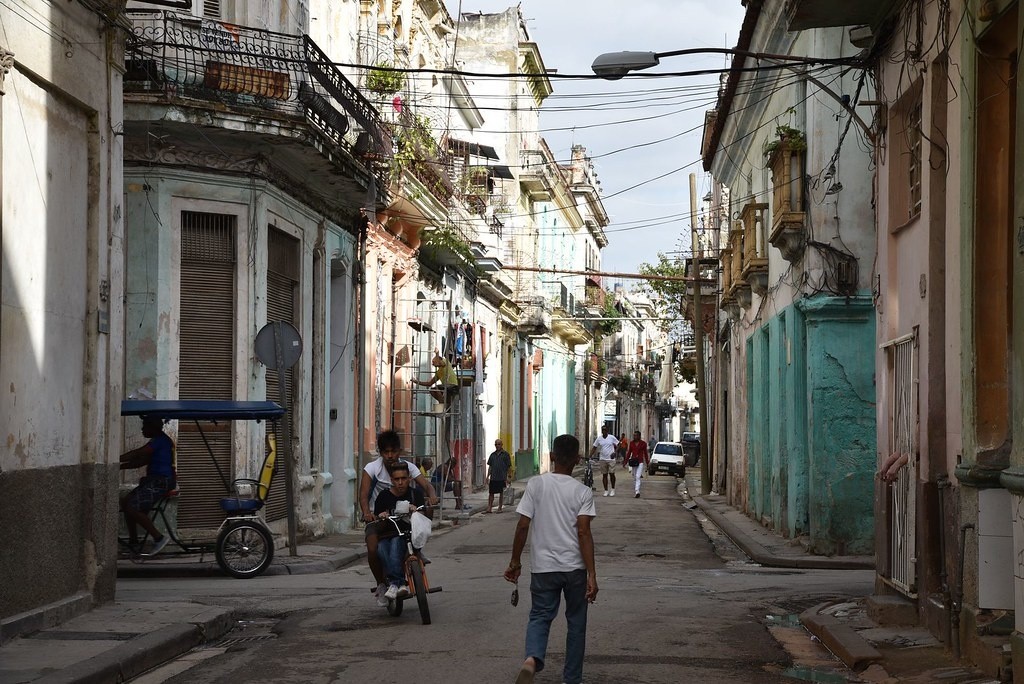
[682,437,700,467]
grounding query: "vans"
[682,432,701,444]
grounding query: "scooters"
[369,497,442,625]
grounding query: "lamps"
[589,48,876,145]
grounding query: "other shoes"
[149,536,171,556]
[120,544,143,555]
[482,511,492,514]
[435,504,446,510]
[633,493,640,498]
[497,510,503,513]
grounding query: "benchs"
[221,432,278,509]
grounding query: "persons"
[120,416,176,557]
[590,425,621,497]
[431,457,471,510]
[359,430,438,606]
[503,435,599,684]
[420,458,433,477]
[623,430,649,498]
[410,346,458,404]
[619,432,627,457]
[648,435,657,458]
[374,462,426,599]
[483,439,512,514]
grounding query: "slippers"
[516,664,535,684]
[454,503,472,510]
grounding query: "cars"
[648,442,687,477]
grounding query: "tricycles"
[119,401,288,580]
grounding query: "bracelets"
[509,563,522,569]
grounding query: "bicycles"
[581,457,599,488]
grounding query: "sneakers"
[418,552,431,563]
[385,584,398,600]
[397,585,408,597]
[610,488,615,496]
[375,583,390,607]
[603,491,609,496]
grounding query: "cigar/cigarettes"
[592,601,596,603]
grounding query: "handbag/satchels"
[629,459,639,466]
[411,510,433,548]
